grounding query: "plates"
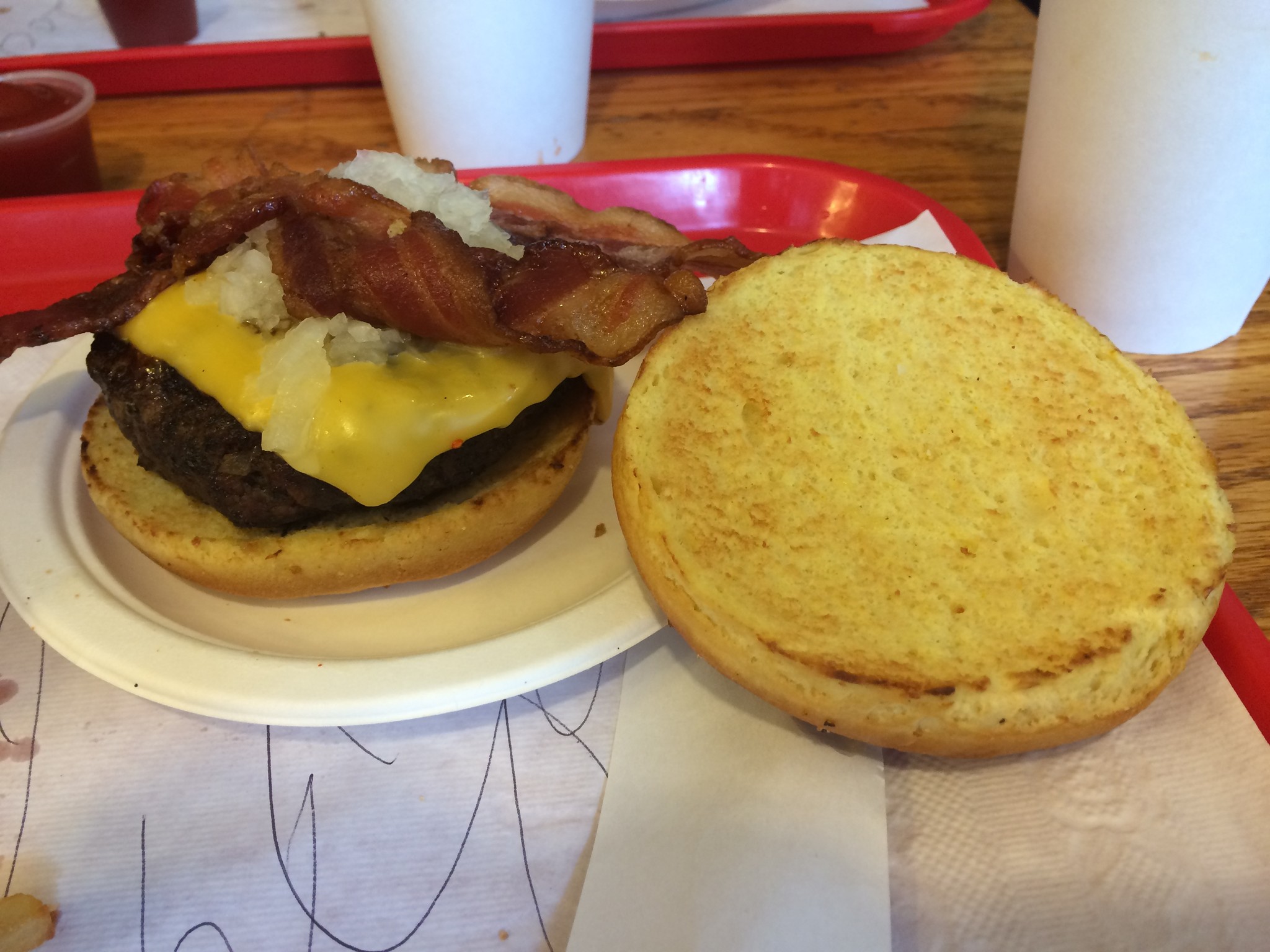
[5,326,673,731]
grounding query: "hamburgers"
[0,151,1237,764]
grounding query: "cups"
[1010,2,1270,353]
[0,65,101,196]
[357,0,594,168]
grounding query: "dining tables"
[0,0,1270,952]
